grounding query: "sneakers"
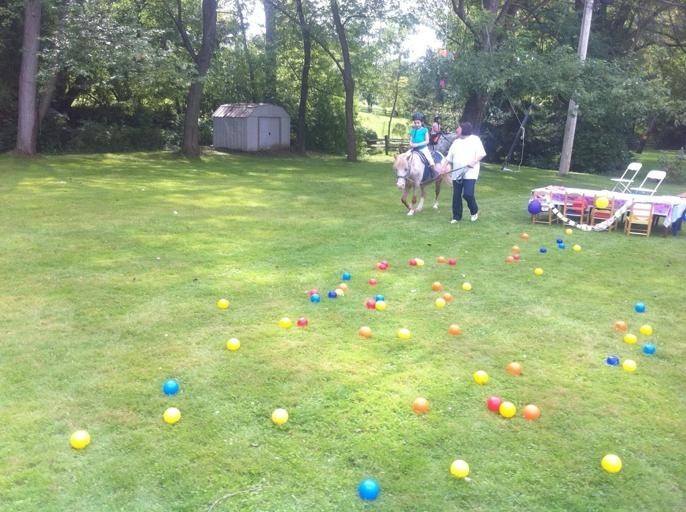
[471,213,477,221]
[451,220,457,224]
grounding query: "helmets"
[435,114,441,119]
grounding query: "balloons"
[596,196,609,209]
[573,197,589,214]
[528,200,542,215]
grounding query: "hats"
[411,112,425,121]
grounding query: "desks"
[529,185,684,238]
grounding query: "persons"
[430,115,443,144]
[442,120,487,224]
[408,112,438,177]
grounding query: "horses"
[391,148,453,217]
[423,130,458,158]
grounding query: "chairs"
[531,191,654,238]
[608,163,666,196]
